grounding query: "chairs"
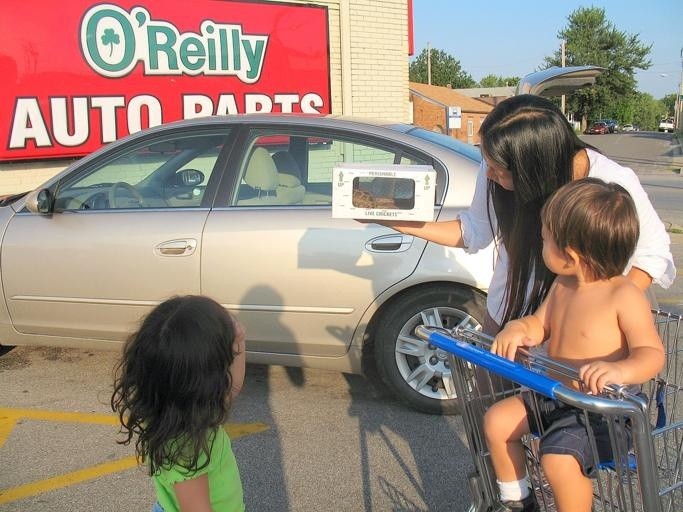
[236,147,305,206]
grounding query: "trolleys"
[416,306,680,512]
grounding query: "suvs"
[659,119,674,133]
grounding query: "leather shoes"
[488,488,540,512]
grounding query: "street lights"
[660,73,680,129]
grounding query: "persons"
[483,177,665,512]
[354,95,677,393]
[101,294,250,512]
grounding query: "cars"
[606,120,620,132]
[585,122,610,135]
[0,110,519,418]
[623,124,639,133]
